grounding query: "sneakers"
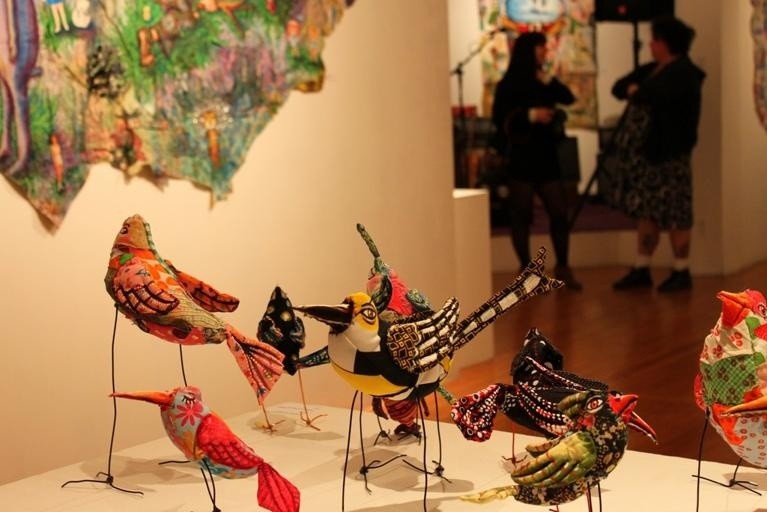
[554,266,582,291]
[612,266,653,290]
[657,267,690,291]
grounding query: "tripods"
[570,23,649,227]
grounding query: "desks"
[452,187,498,370]
[0,399,765,511]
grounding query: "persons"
[599,15,706,294]
[489,30,586,292]
